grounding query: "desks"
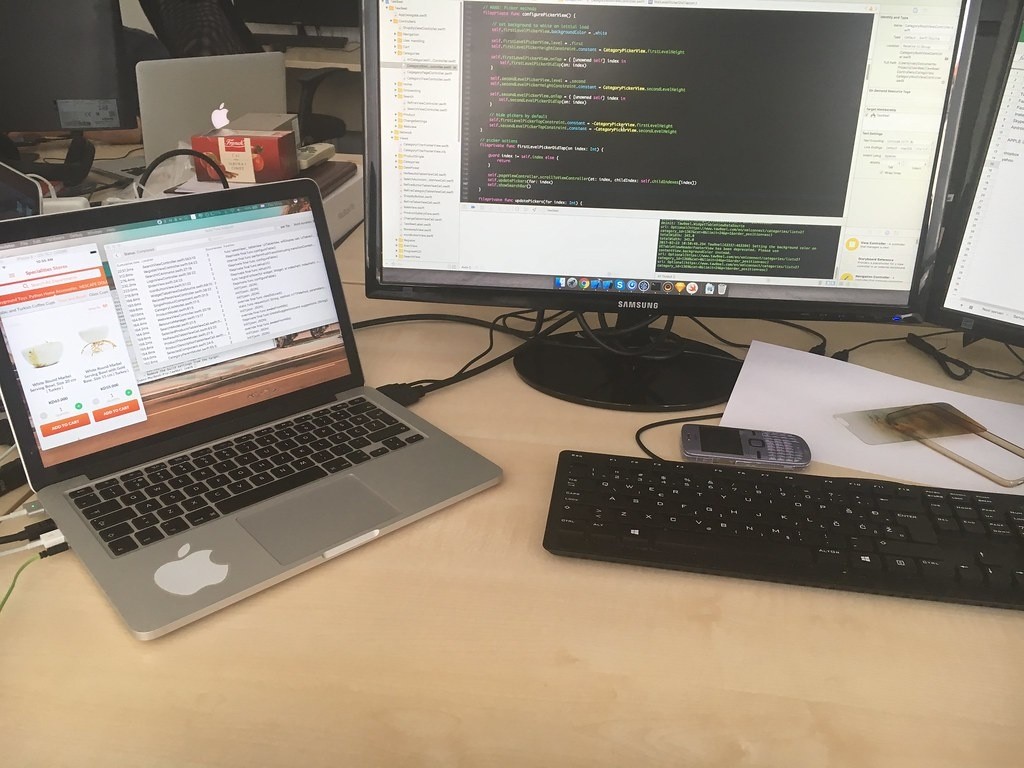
[0,142,1024,767]
[258,41,362,76]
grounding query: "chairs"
[138,0,348,147]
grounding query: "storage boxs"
[191,128,299,183]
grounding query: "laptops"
[137,51,285,172]
[0,176,503,642]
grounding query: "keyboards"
[542,449,1024,613]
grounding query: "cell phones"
[679,424,811,472]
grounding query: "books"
[162,161,357,206]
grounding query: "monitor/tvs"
[924,0,1024,351]
[361,0,983,414]
[0,0,138,162]
